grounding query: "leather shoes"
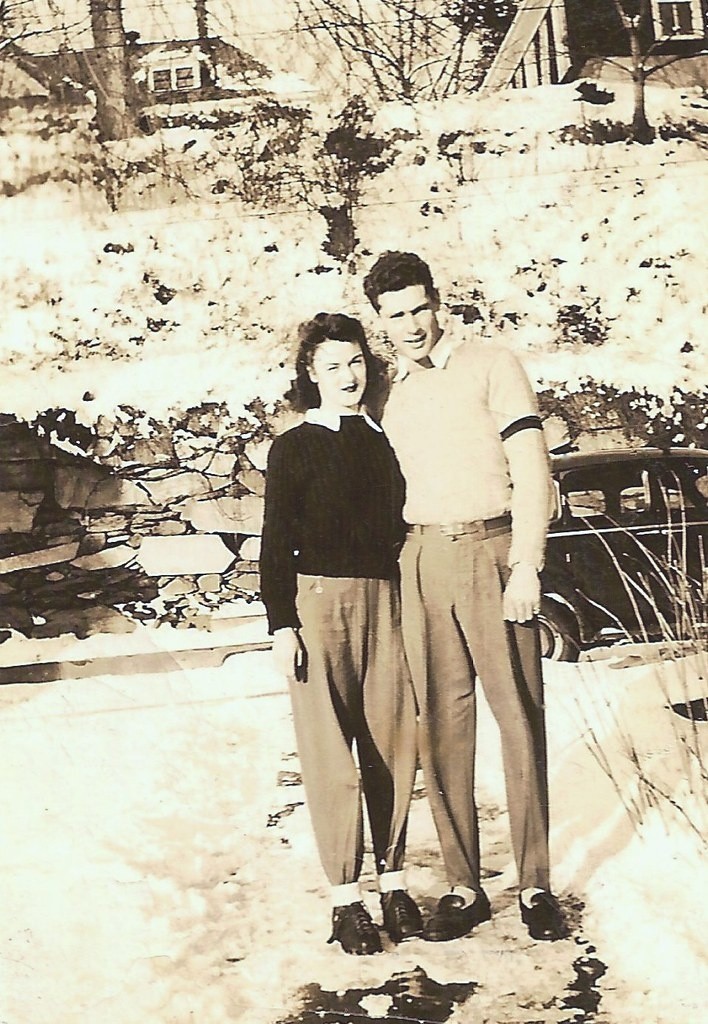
[327,901,382,955]
[429,889,490,939]
[518,890,566,940]
[380,890,423,940]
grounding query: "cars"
[529,448,708,663]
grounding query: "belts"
[405,514,512,536]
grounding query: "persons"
[363,252,566,942]
[257,312,424,959]
